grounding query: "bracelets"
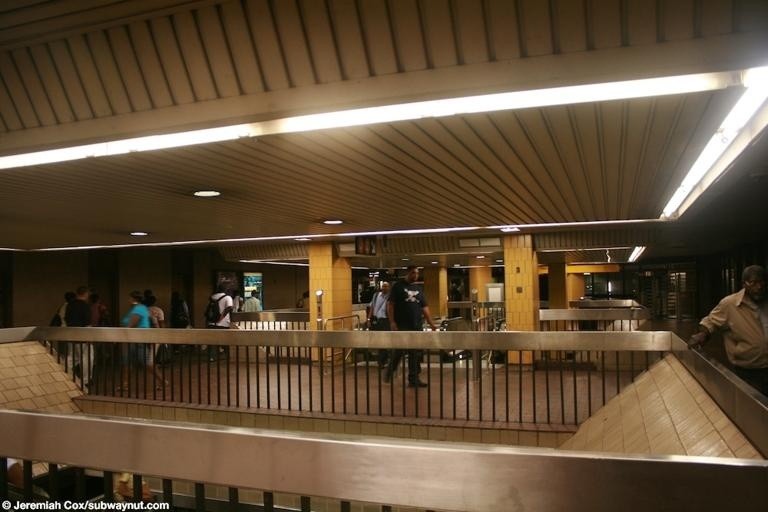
[367,319,370,321]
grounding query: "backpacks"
[205,293,230,324]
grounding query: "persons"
[88,292,110,371]
[367,280,393,369]
[298,291,309,308]
[56,292,76,358]
[450,284,461,317]
[145,295,169,379]
[141,289,153,306]
[171,291,191,354]
[66,286,95,387]
[687,264,768,394]
[115,289,169,392]
[383,266,436,387]
[204,284,262,364]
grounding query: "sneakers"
[382,370,428,388]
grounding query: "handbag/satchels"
[366,305,373,320]
